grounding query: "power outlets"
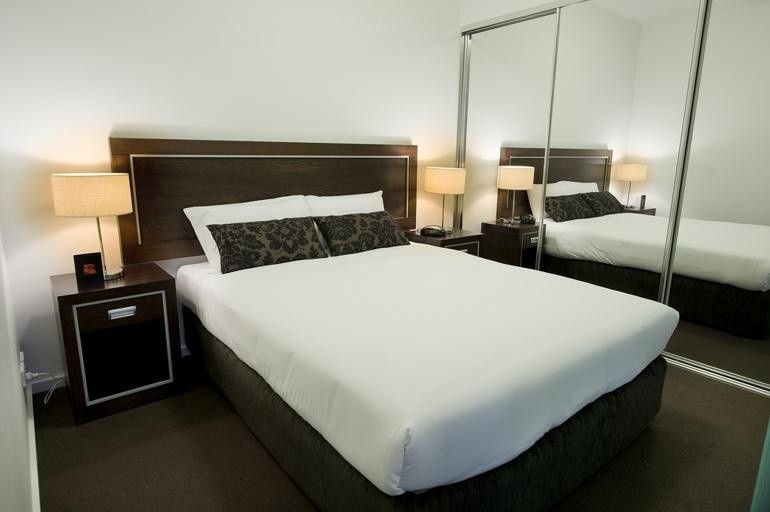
[19,351,28,388]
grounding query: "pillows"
[527,182,568,219]
[559,180,600,195]
[206,216,330,273]
[313,211,411,257]
[545,195,595,223]
[183,195,328,275]
[306,190,384,216]
[582,192,625,216]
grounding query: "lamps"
[498,166,536,224]
[424,166,467,235]
[615,163,647,208]
[51,173,135,282]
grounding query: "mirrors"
[536,0,707,304]
[453,0,559,270]
[658,0,769,399]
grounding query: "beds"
[500,148,769,342]
[111,137,681,512]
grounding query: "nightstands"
[404,224,485,258]
[481,221,546,267]
[623,204,656,216]
[50,263,184,427]
